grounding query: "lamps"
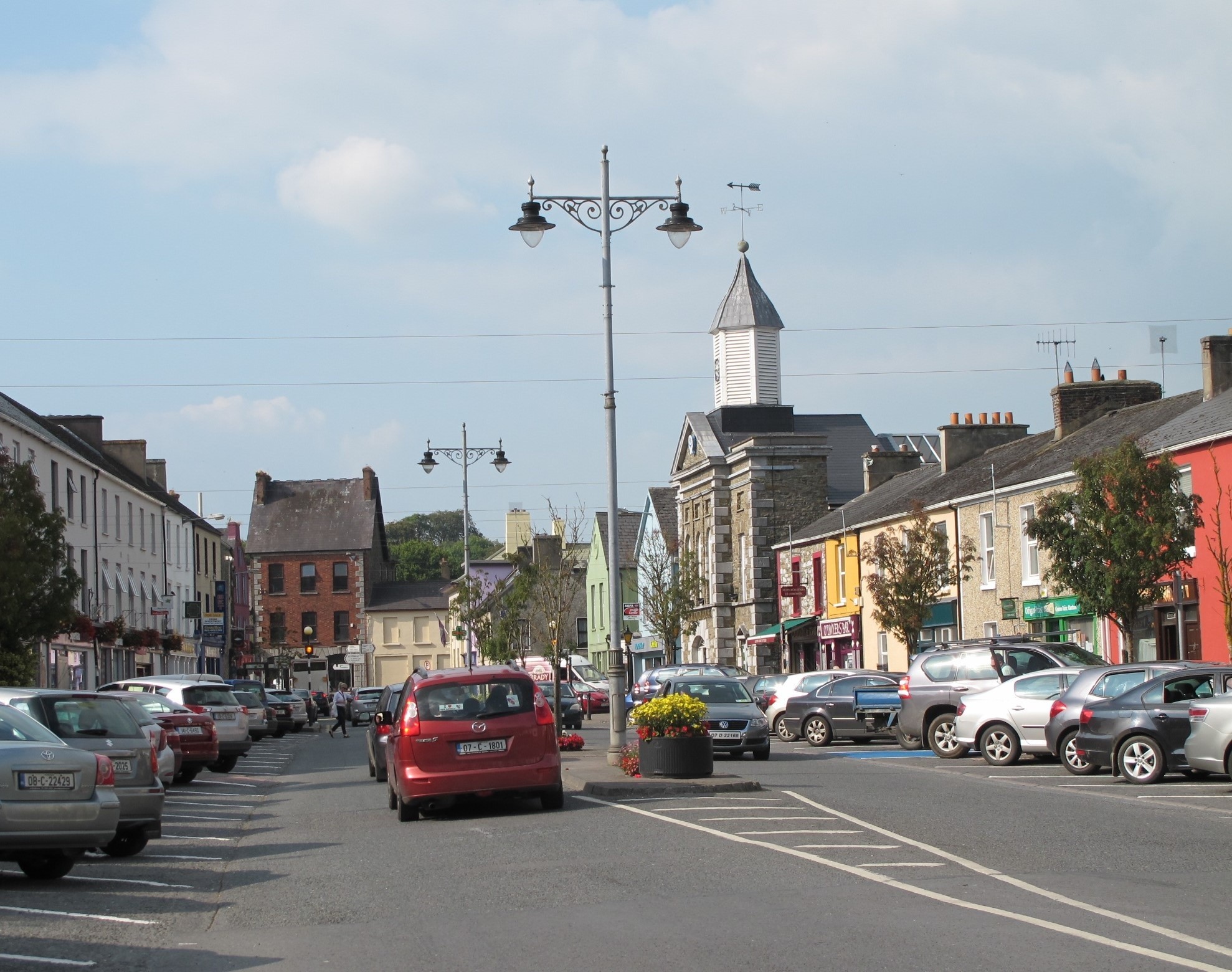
[232,568,260,577]
[727,593,737,600]
[182,514,225,526]
[696,597,705,604]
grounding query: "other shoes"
[329,729,334,738]
[344,735,350,738]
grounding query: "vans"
[512,653,612,714]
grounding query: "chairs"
[708,687,719,694]
[462,699,481,713]
[1163,688,1172,703]
[1027,656,1046,671]
[1009,655,1018,672]
[1174,682,1195,698]
[78,708,97,729]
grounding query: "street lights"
[304,626,313,695]
[506,147,703,770]
[420,420,510,670]
[623,624,634,695]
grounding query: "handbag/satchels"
[345,702,351,720]
[331,703,338,718]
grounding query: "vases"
[636,734,715,778]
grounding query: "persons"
[465,685,508,717]
[377,716,383,723]
[328,681,355,738]
[990,654,1016,685]
[680,686,692,696]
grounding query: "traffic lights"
[305,644,313,656]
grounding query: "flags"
[436,614,446,647]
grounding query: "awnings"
[747,617,814,646]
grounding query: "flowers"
[627,692,711,741]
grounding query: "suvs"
[1044,658,1228,777]
[896,628,1109,760]
[763,667,885,744]
[0,687,167,858]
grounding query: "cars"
[1074,665,1232,784]
[534,680,583,729]
[374,659,565,821]
[51,673,331,790]
[626,662,799,726]
[652,675,771,760]
[783,672,923,748]
[953,665,1104,766]
[344,686,388,726]
[0,701,122,885]
[358,682,410,783]
[1182,692,1232,783]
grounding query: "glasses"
[344,688,347,690]
